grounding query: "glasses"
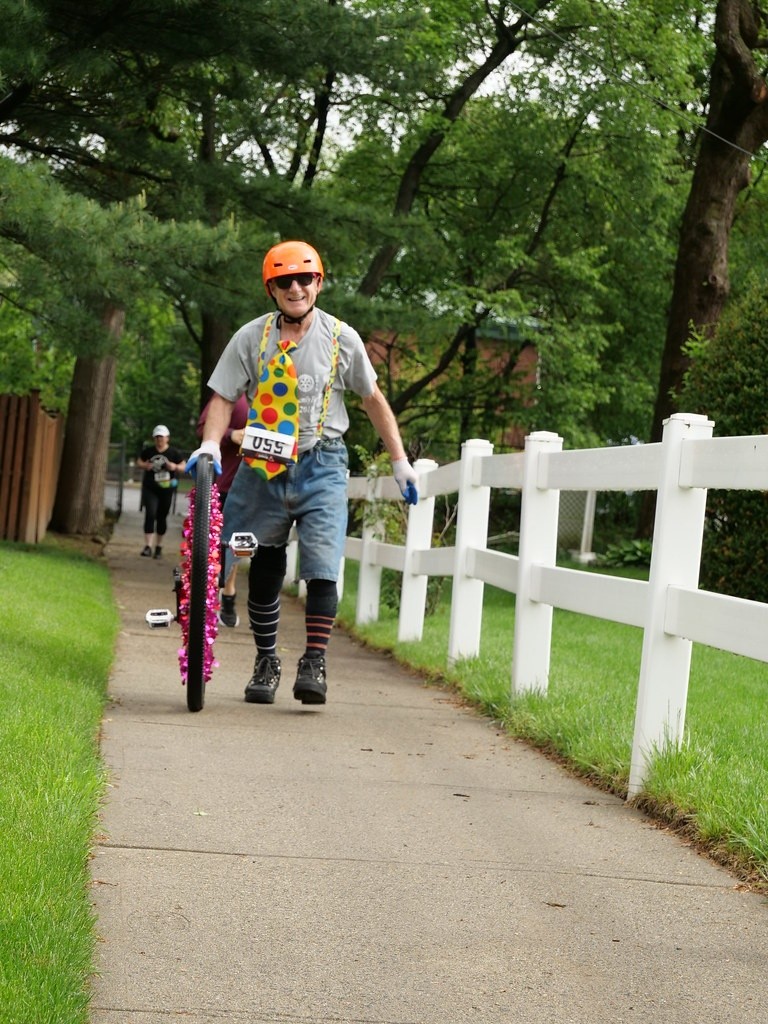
[267,272,317,290]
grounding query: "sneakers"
[216,588,240,628]
[244,654,282,704]
[141,546,151,556]
[293,654,328,705]
[153,546,162,559]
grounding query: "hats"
[152,424,171,438]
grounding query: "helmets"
[262,240,324,288]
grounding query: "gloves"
[391,457,420,506]
[184,440,222,481]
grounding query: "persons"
[197,392,250,627]
[137,424,186,560]
[183,240,420,700]
